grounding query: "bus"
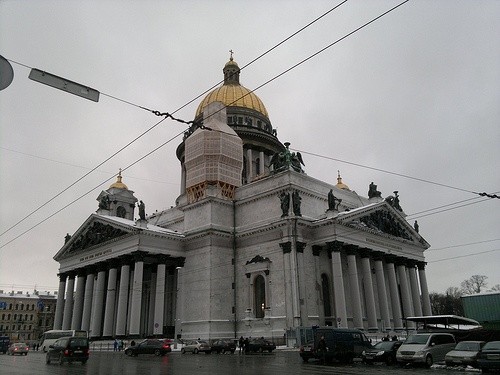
[39,330,89,353]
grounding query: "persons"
[276,152,304,173]
[328,189,336,209]
[381,335,390,341]
[292,189,302,217]
[33,343,36,351]
[137,200,145,220]
[243,338,250,354]
[120,339,124,350]
[367,181,377,198]
[414,220,419,233]
[238,336,244,351]
[64,233,71,244]
[114,340,118,351]
[278,189,290,217]
[37,343,39,351]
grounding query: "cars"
[299,340,318,362]
[445,341,485,369]
[8,343,28,356]
[241,339,276,353]
[180,340,212,354]
[205,338,237,354]
[124,339,171,356]
[474,341,500,373]
[361,341,404,366]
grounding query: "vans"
[0,336,10,355]
[396,333,457,367]
[311,326,374,364]
[46,337,90,366]
